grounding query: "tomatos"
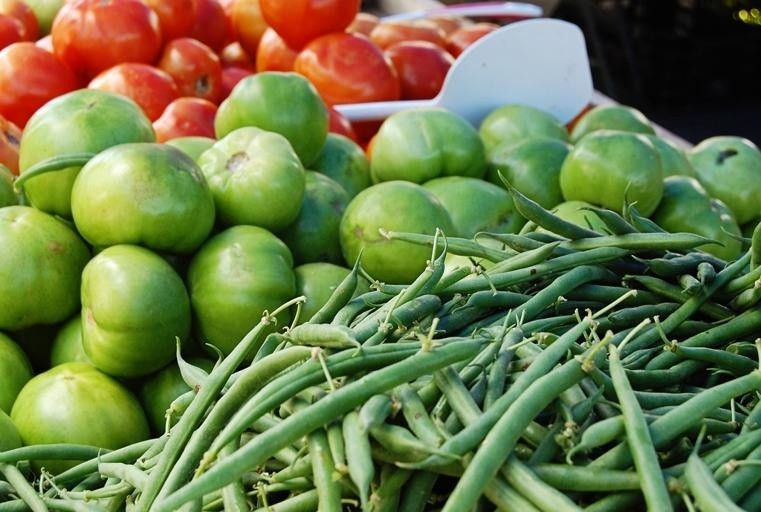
[0,0,515,176]
[0,71,761,480]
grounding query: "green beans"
[0,167,761,512]
